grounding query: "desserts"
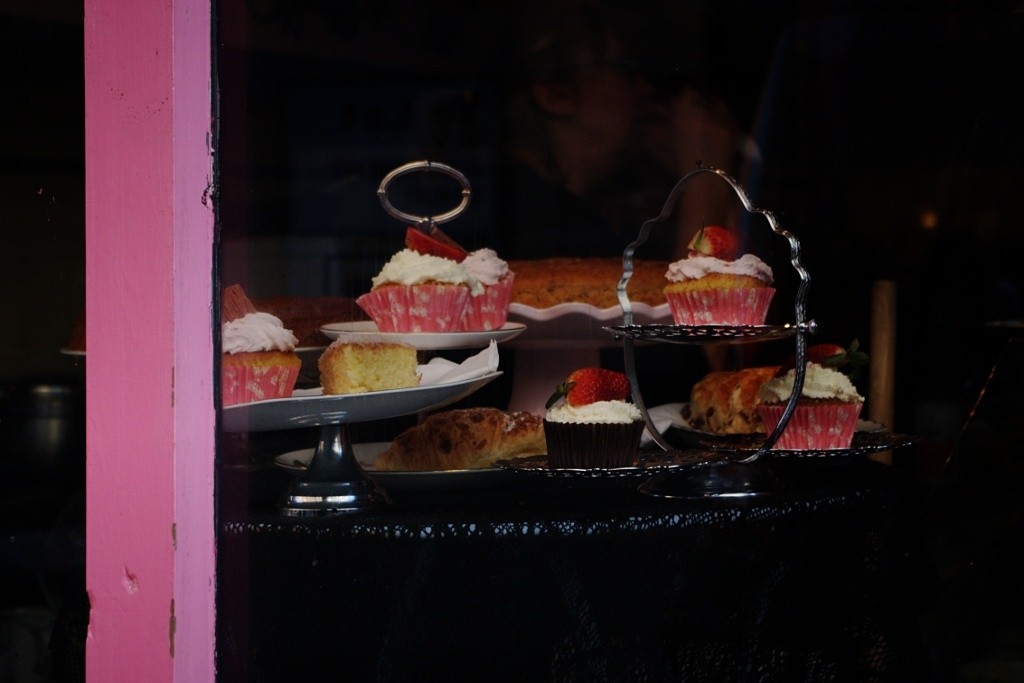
[222,208,862,478]
